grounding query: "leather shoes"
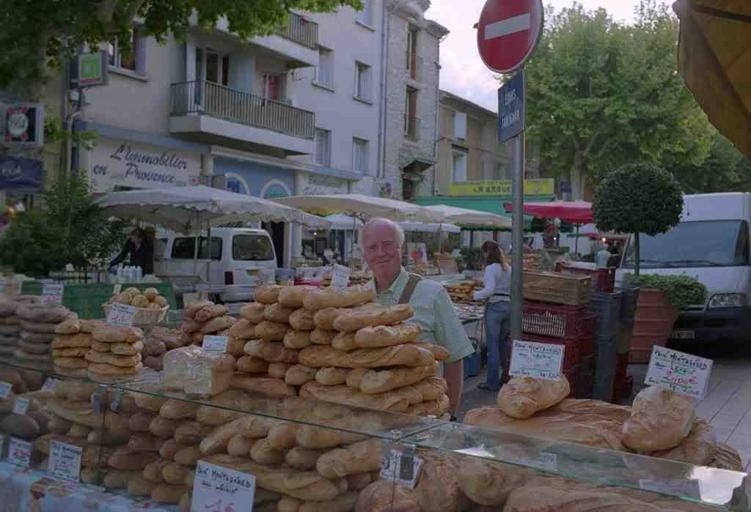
[476,382,488,390]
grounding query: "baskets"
[503,262,640,406]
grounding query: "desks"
[19,279,177,321]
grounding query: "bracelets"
[450,415,458,422]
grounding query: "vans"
[613,192,751,343]
[152,227,278,310]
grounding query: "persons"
[108,227,153,276]
[469,240,513,391]
[145,224,171,260]
[594,238,625,268]
[360,216,476,422]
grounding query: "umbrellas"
[503,197,596,256]
[95,182,331,284]
[268,194,514,266]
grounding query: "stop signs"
[477,0,544,74]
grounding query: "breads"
[0,269,747,512]
